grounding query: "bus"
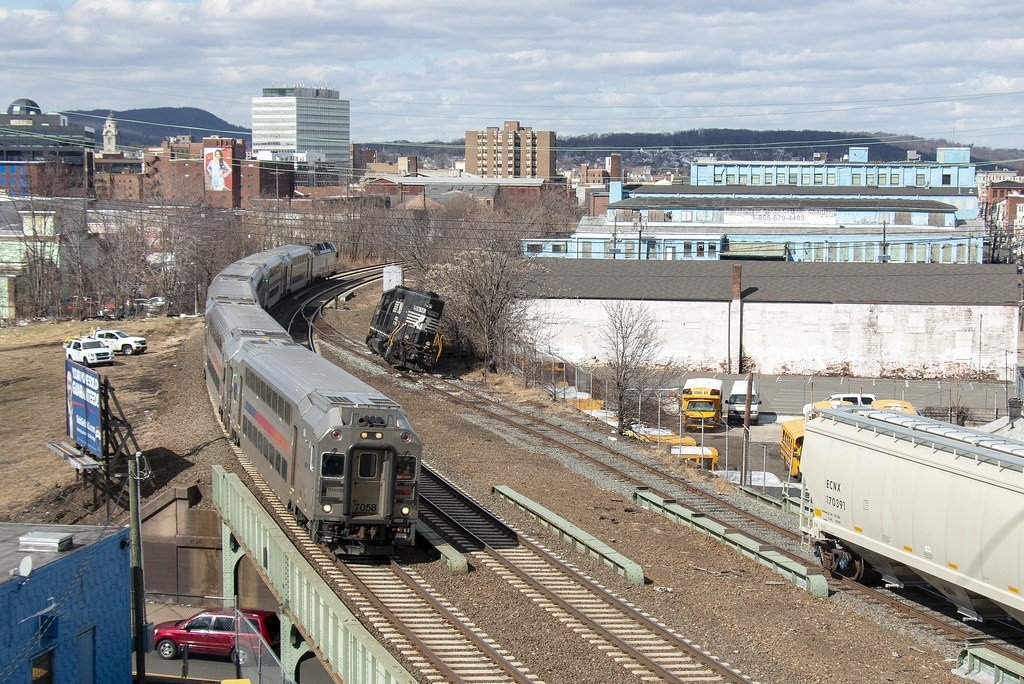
[682,378,723,432]
[781,419,808,482]
[535,358,718,471]
[870,399,920,416]
[811,401,855,421]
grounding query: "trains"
[205,239,422,563]
[799,404,1024,643]
[366,286,445,374]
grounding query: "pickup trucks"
[63,329,147,356]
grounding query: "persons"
[207,150,232,191]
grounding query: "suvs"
[66,338,115,368]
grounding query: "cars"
[804,393,875,417]
[65,294,174,321]
[154,609,281,667]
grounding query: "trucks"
[725,379,763,425]
